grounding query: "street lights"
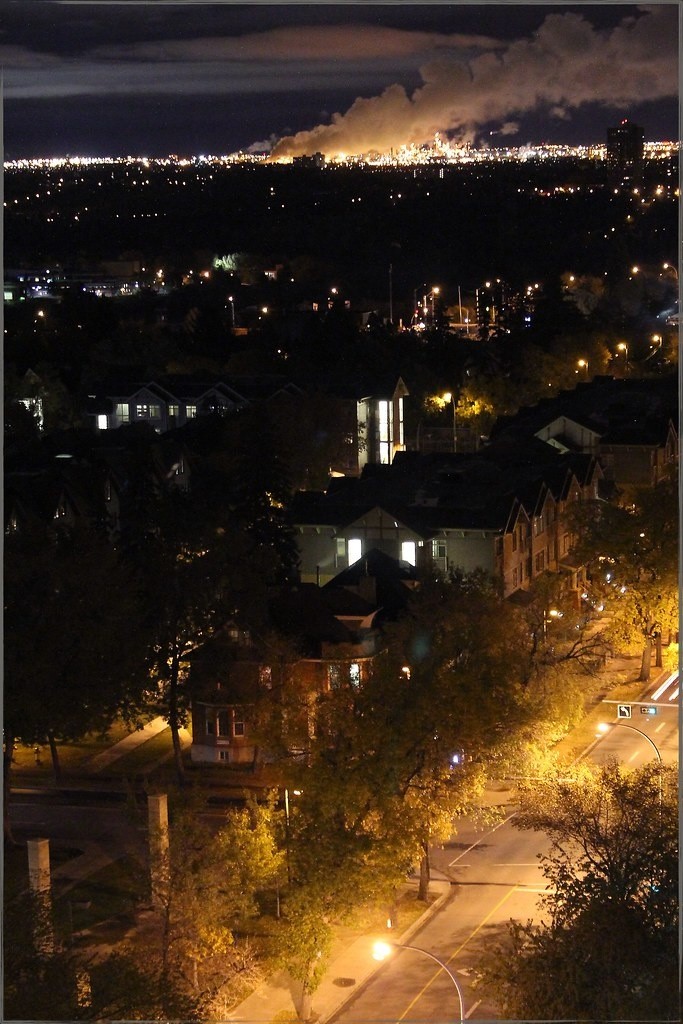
[653,335,663,348]
[423,286,440,317]
[372,940,464,1024]
[598,722,663,827]
[578,359,589,375]
[619,343,628,361]
[663,264,677,278]
[444,393,457,452]
[476,282,491,321]
[543,610,557,645]
[285,788,303,890]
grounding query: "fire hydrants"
[34,748,41,762]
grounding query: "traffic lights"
[640,707,656,714]
